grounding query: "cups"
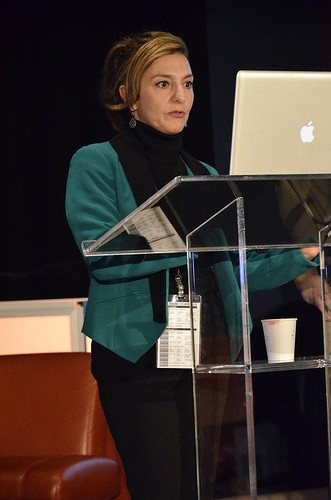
[261,318,297,364]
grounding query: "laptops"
[228,70,331,176]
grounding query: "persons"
[65,30,322,500]
[280,179,331,485]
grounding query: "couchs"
[0,352,132,500]
